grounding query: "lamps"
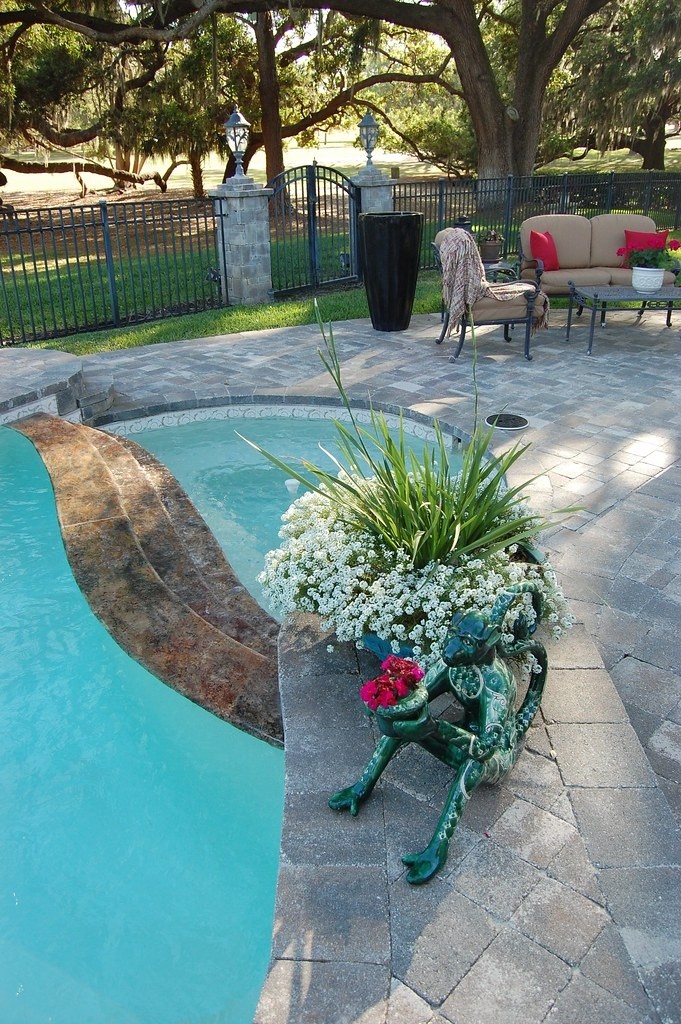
[224,103,251,165]
[357,109,380,164]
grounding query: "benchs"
[511,214,681,329]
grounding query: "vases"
[632,267,665,294]
[358,211,424,332]
[352,541,557,661]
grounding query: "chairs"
[431,228,549,363]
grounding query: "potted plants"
[477,230,505,258]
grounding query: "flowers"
[616,240,681,268]
[232,294,577,674]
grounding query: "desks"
[565,280,681,356]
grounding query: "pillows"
[622,229,669,268]
[530,229,561,270]
